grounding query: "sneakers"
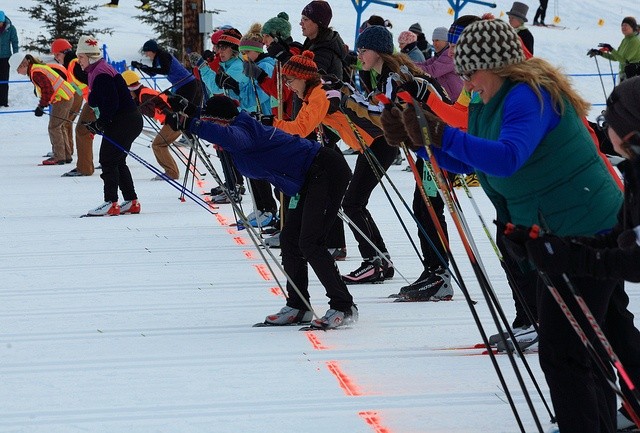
[155,173,173,179]
[341,257,384,284]
[489,328,522,345]
[454,173,480,188]
[310,305,358,330]
[379,253,394,280]
[328,248,346,260]
[498,324,540,354]
[212,190,242,204]
[539,21,546,26]
[400,268,454,302]
[249,212,280,228]
[88,201,120,216]
[43,158,64,165]
[61,168,83,176]
[266,307,312,326]
[532,20,539,25]
[211,182,245,196]
[119,200,140,214]
[245,210,262,222]
[264,231,280,248]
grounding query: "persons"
[74,34,144,215]
[408,23,432,61]
[594,72,639,244]
[242,12,304,248]
[257,50,401,285]
[7,51,74,165]
[397,31,425,64]
[162,92,358,331]
[531,0,549,27]
[187,28,258,204]
[378,16,640,433]
[0,10,18,108]
[40,65,83,159]
[49,39,99,177]
[192,49,221,79]
[319,16,455,301]
[412,28,465,104]
[287,0,345,80]
[189,25,232,80]
[587,16,640,84]
[214,23,277,228]
[358,15,393,33]
[131,39,201,144]
[121,70,182,182]
[396,14,495,132]
[505,1,535,55]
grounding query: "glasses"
[283,78,297,88]
[457,72,476,82]
[217,46,229,51]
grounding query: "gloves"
[167,113,189,132]
[586,48,602,57]
[326,88,346,106]
[35,106,44,116]
[401,80,429,101]
[321,74,343,90]
[215,67,240,96]
[380,106,409,145]
[85,122,101,135]
[130,61,141,68]
[529,227,607,275]
[242,56,262,79]
[168,95,193,114]
[187,52,206,68]
[252,111,275,126]
[597,43,613,52]
[499,226,538,259]
[403,104,448,149]
[145,67,157,76]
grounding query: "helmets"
[9,53,26,74]
[121,70,139,85]
[51,39,72,52]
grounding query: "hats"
[75,35,100,55]
[447,15,482,45]
[211,25,234,45]
[0,10,5,22]
[142,40,157,51]
[605,76,639,157]
[506,2,529,22]
[410,22,422,33]
[238,24,263,53]
[261,12,291,40]
[301,0,332,27]
[200,96,240,123]
[65,157,72,163]
[398,30,417,44]
[454,13,526,73]
[218,28,242,50]
[367,15,386,28]
[433,27,448,42]
[282,50,318,80]
[356,26,394,54]
[621,17,639,33]
